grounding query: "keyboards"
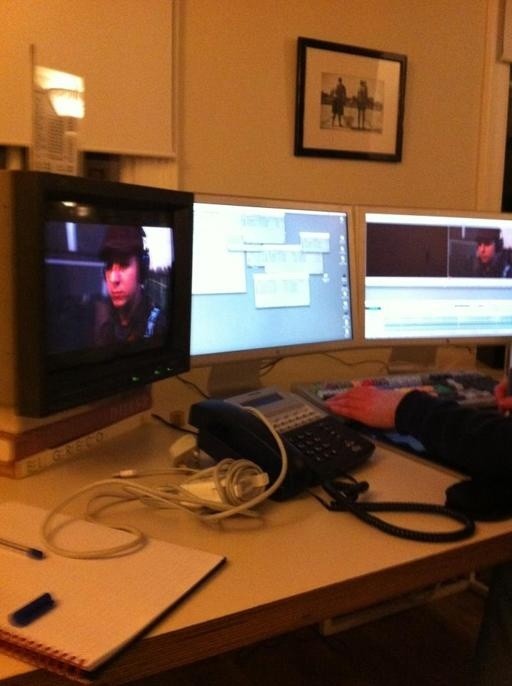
[292,374,501,424]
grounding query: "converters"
[180,475,252,508]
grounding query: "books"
[0,502,226,682]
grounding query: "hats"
[476,229,500,244]
[97,226,149,261]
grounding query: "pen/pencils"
[0,537,44,559]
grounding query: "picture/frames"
[296,36,409,165]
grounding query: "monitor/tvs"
[190,192,360,400]
[0,169,194,418]
[353,205,512,377]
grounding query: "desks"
[0,347,512,685]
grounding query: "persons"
[472,229,511,278]
[94,223,169,345]
[356,81,366,128]
[331,77,349,129]
[326,368,512,492]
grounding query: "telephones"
[189,386,375,501]
